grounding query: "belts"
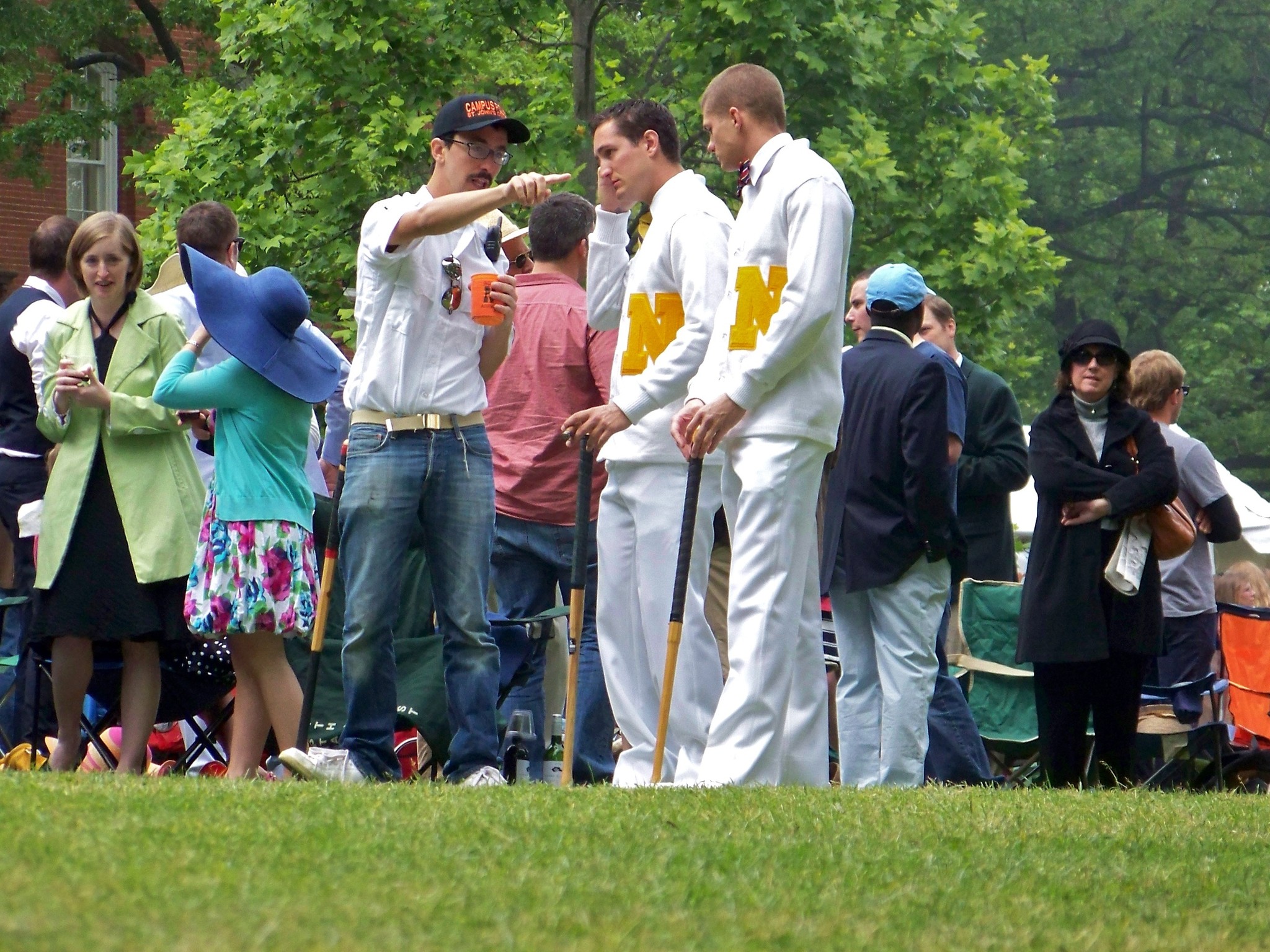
[350,410,485,432]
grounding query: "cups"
[470,273,504,326]
[505,709,537,741]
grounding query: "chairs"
[30,491,569,782]
[945,578,1270,790]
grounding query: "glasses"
[441,136,513,166]
[227,237,244,251]
[441,254,462,314]
[510,251,534,268]
[1073,349,1117,366]
[1178,385,1190,395]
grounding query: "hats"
[432,95,530,144]
[475,208,530,242]
[180,244,340,403]
[1057,320,1130,373]
[866,262,937,314]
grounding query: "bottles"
[543,714,564,786]
[503,714,530,785]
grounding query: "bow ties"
[735,159,751,197]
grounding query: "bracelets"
[187,340,201,351]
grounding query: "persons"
[561,100,734,787]
[1216,559,1270,724]
[1128,349,1241,783]
[477,207,571,747]
[1016,319,1180,788]
[153,243,341,780]
[845,271,994,786]
[824,263,969,788]
[671,65,854,787]
[33,212,205,776]
[0,215,81,755]
[919,295,1030,774]
[153,201,351,779]
[279,97,570,786]
[482,192,618,787]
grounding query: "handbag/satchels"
[1126,438,1196,560]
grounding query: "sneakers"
[278,746,366,783]
[460,765,507,786]
